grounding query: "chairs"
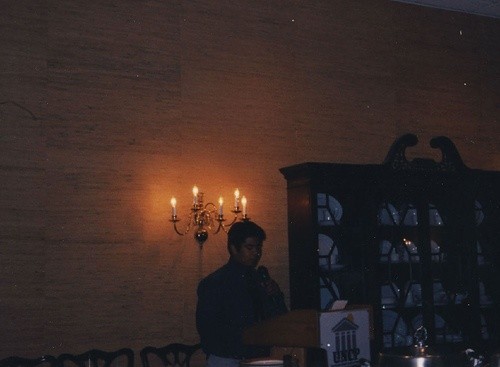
[0,342,207,367]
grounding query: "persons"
[196,222,289,367]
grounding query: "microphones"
[258,266,276,303]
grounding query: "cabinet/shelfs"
[279,134,500,367]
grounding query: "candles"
[169,183,248,219]
